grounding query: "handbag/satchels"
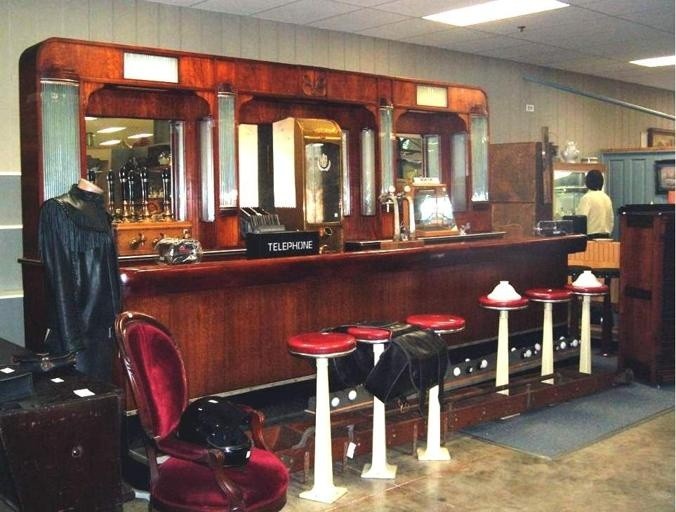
[12,351,77,371]
[313,318,452,416]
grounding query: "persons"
[573,169,615,239]
[37,178,124,390]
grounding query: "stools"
[477,294,529,397]
[566,265,589,346]
[525,287,572,407]
[287,333,357,504]
[590,266,620,357]
[405,314,465,463]
[565,284,610,374]
[342,325,399,480]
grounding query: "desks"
[0,337,126,511]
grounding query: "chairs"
[112,310,289,512]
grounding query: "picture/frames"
[647,126,674,148]
[654,158,675,194]
